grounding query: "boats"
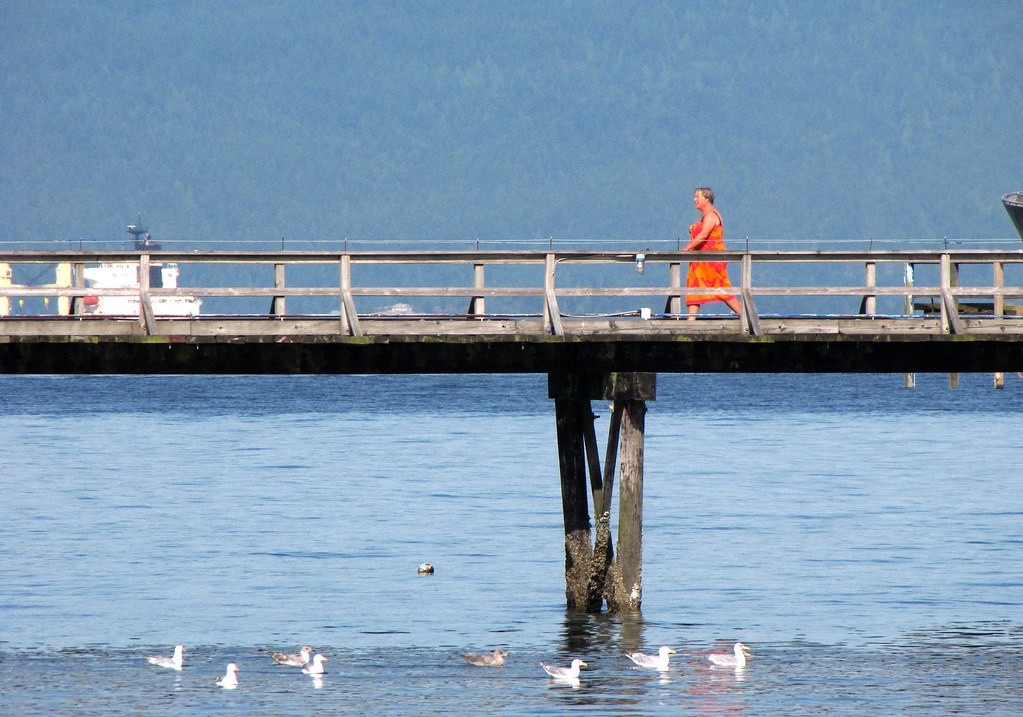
[67,211,204,320]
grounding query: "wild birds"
[538,659,587,678]
[456,648,510,667]
[266,646,329,675]
[624,645,676,669]
[215,663,240,688]
[144,645,187,672]
[702,642,751,668]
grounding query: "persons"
[676,186,744,321]
